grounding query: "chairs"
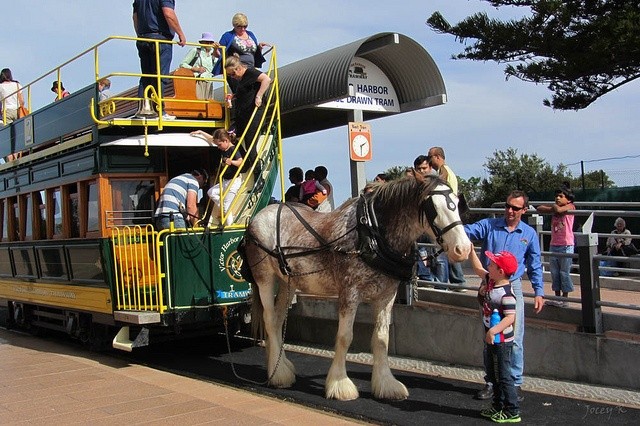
[157,113,176,121]
[552,301,568,307]
[448,287,467,292]
[491,410,521,423]
[545,299,558,305]
[480,407,496,417]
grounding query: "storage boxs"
[99,67,223,118]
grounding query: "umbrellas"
[514,386,525,402]
[476,384,494,399]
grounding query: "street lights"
[17,107,29,118]
[599,260,619,276]
[622,243,638,255]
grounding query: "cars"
[225,94,233,108]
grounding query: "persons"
[98,79,110,101]
[606,218,632,276]
[179,32,219,100]
[51,81,70,101]
[427,147,468,292]
[153,167,209,233]
[413,155,449,289]
[225,53,271,193]
[537,188,576,308]
[191,129,243,226]
[0,68,24,124]
[465,241,522,423]
[211,13,271,94]
[133,0,186,120]
[376,174,388,183]
[305,166,334,213]
[299,170,327,202]
[464,190,545,402]
[281,168,303,202]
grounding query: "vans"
[248,177,265,193]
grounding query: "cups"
[237,26,248,29]
[504,203,523,211]
[203,182,207,186]
[227,72,236,76]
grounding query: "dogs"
[244,165,473,403]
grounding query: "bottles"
[199,33,214,43]
[485,250,518,276]
[51,81,65,92]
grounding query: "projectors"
[0,35,473,402]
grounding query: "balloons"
[348,122,372,163]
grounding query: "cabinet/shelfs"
[490,309,500,343]
